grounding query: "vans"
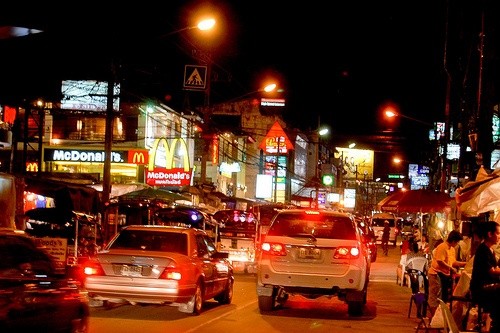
[256,209,372,318]
[357,213,404,262]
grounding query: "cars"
[80,225,236,315]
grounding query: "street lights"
[201,82,276,184]
[102,15,218,250]
[385,108,446,193]
[285,129,329,206]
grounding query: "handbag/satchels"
[430,304,446,327]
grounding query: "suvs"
[0,229,89,333]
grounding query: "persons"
[380,220,500,333]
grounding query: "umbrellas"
[378,187,455,244]
[117,188,197,224]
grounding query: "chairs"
[395,254,459,333]
